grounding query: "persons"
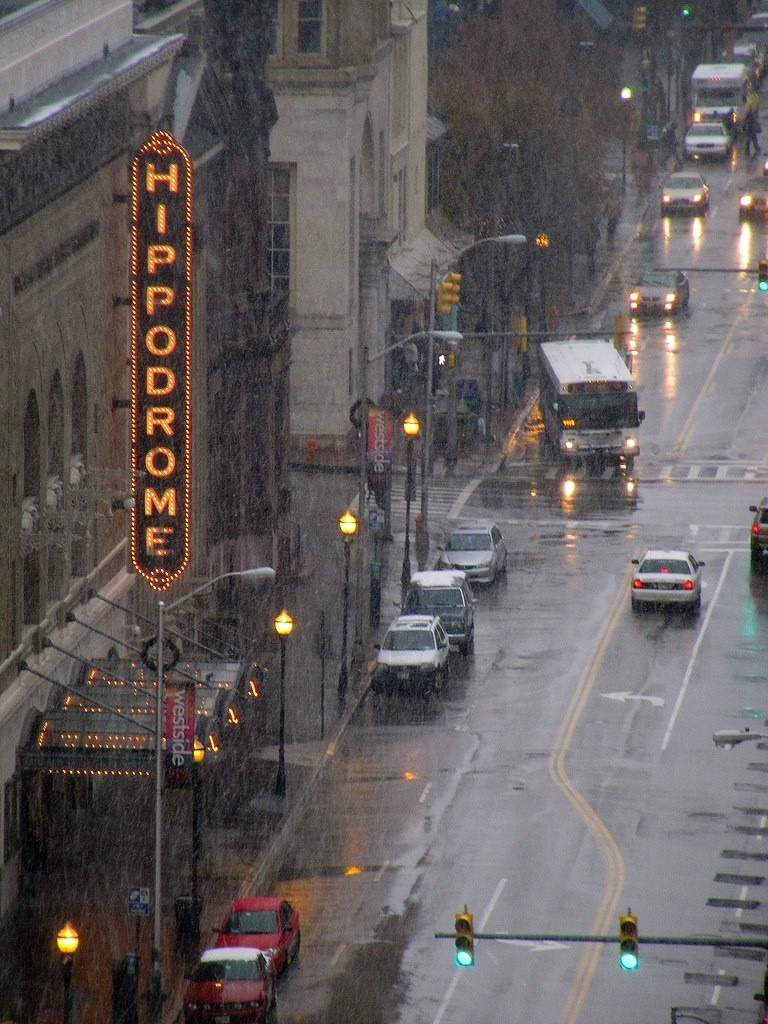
[349,639,363,698]
[743,106,761,154]
[660,122,684,169]
[603,190,621,252]
[724,109,737,154]
[712,110,721,119]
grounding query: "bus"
[538,342,646,466]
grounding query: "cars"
[215,897,301,975]
[404,568,480,661]
[183,945,278,1024]
[372,614,454,694]
[625,10,768,618]
[437,523,510,593]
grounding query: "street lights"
[358,330,462,523]
[401,412,419,586]
[149,566,276,997]
[414,235,524,526]
[338,510,359,692]
[621,86,633,195]
[275,606,292,795]
[57,922,81,1024]
[187,739,205,942]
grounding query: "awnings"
[389,217,475,301]
[14,656,244,790]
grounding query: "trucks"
[689,62,749,132]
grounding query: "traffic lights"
[618,915,639,970]
[437,271,461,314]
[454,913,474,967]
